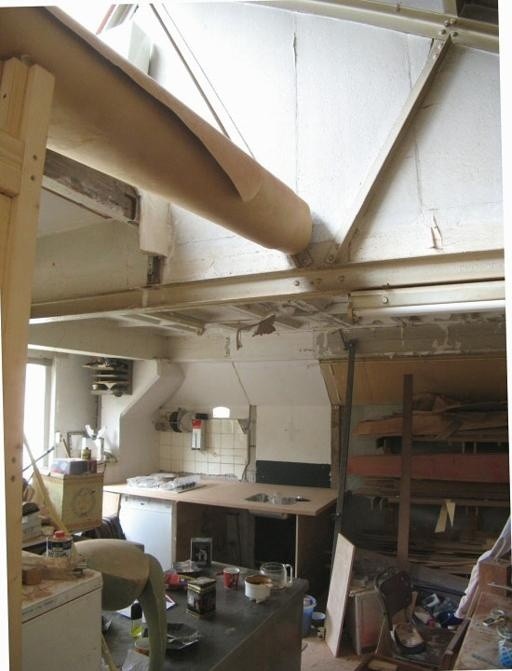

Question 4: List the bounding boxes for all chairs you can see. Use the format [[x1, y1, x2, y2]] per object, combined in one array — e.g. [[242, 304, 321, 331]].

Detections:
[[373, 564, 460, 671]]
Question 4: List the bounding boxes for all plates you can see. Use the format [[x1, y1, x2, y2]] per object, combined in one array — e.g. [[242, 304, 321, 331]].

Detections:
[[170, 411, 194, 433]]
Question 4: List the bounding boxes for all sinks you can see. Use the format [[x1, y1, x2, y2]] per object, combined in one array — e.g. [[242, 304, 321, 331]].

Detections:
[[245, 493, 310, 521]]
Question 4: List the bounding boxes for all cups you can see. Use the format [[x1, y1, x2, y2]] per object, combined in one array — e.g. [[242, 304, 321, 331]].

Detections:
[[135, 638, 149, 658], [260, 562, 293, 589], [245, 575, 271, 603], [223, 567, 241, 590], [89, 461, 97, 474]]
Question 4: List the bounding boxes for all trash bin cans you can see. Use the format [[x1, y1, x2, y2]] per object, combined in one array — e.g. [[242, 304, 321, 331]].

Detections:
[[298, 595, 318, 640]]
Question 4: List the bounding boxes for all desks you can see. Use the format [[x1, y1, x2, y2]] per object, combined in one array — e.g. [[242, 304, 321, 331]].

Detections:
[[94, 550, 309, 671]]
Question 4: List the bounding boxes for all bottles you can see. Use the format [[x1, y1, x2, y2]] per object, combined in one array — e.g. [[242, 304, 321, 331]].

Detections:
[[130, 604, 142, 637], [46, 529, 74, 560], [192, 419, 202, 451], [81, 447, 92, 463]]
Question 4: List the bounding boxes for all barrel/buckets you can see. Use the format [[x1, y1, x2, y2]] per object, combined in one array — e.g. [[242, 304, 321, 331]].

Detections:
[[300, 594, 316, 638]]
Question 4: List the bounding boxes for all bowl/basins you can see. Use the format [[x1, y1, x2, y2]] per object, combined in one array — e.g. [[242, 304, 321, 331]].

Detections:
[[151, 472, 179, 482]]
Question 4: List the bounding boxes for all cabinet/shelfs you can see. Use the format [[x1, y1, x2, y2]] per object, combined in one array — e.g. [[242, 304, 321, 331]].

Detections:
[[122, 496, 224, 573]]
